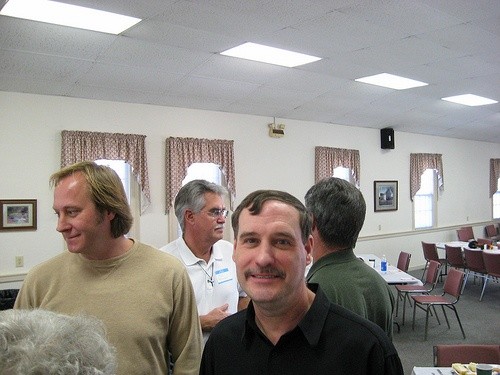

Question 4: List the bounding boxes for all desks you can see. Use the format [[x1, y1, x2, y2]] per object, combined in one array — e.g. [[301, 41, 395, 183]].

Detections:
[[366, 260, 423, 334], [436, 241, 500, 254]]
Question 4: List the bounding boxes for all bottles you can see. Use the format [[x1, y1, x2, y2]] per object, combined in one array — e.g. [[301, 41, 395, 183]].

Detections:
[[369, 256, 375, 269], [380, 254, 388, 275]]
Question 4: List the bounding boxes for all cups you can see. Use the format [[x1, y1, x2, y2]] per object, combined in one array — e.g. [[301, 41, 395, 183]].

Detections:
[[476, 364, 493, 375]]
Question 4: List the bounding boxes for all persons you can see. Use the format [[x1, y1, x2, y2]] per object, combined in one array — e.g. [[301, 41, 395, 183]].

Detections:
[[0, 309, 116, 375], [199, 189, 404, 375], [160, 180, 251, 375], [304, 176, 395, 340], [13, 160, 203, 375]]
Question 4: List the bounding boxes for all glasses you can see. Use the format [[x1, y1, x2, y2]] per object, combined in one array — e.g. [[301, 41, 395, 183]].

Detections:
[[192, 209, 229, 219]]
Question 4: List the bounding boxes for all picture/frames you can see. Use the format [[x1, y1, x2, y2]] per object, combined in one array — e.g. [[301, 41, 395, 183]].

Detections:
[[0, 199, 37, 230], [374, 181, 398, 212]]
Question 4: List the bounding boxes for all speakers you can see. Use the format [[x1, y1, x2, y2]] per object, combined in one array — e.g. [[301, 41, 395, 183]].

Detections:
[[381, 128, 394, 149]]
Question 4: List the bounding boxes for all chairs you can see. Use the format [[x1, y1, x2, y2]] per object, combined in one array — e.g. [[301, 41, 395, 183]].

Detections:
[[396, 225, 500, 367]]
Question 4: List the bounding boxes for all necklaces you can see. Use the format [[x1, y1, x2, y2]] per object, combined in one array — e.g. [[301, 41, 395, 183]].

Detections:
[[197, 262, 214, 287]]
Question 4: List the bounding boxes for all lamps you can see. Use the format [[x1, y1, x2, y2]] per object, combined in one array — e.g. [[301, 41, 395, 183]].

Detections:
[[268, 118, 286, 138]]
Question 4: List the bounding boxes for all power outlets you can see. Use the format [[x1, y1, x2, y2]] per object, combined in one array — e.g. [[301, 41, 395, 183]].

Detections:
[[16, 256, 24, 267]]
